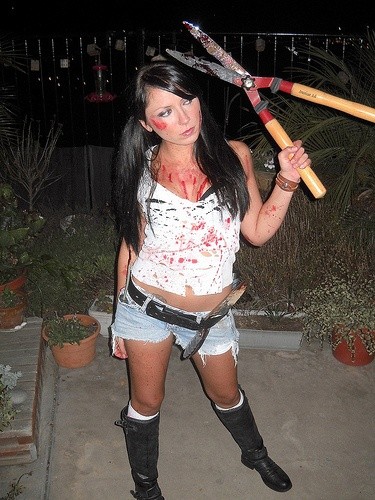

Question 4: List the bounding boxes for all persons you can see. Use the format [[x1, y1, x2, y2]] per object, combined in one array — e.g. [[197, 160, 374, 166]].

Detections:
[[111, 61, 312, 499]]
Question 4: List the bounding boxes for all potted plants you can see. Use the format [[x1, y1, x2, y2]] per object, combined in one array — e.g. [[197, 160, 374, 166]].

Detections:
[[231, 189, 319, 351], [0, 183, 116, 368]]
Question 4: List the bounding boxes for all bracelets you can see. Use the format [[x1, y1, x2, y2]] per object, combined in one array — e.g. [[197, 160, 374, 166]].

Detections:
[[275, 171, 301, 192]]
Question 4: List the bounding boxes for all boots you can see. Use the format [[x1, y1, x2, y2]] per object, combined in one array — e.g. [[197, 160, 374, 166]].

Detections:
[[210, 387, 294, 492], [114, 404, 167, 500]]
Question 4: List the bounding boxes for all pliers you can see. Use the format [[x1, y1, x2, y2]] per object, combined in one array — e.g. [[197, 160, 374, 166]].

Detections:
[[166, 20, 375, 200]]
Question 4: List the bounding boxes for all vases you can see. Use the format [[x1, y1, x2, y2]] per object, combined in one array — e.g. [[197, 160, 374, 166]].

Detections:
[[332, 323, 375, 366]]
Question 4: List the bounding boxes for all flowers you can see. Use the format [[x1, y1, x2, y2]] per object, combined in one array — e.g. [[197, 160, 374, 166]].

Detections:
[[294, 269, 375, 361]]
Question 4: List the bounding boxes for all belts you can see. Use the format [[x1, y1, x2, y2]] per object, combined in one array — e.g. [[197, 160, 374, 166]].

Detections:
[[128, 270, 229, 331]]
